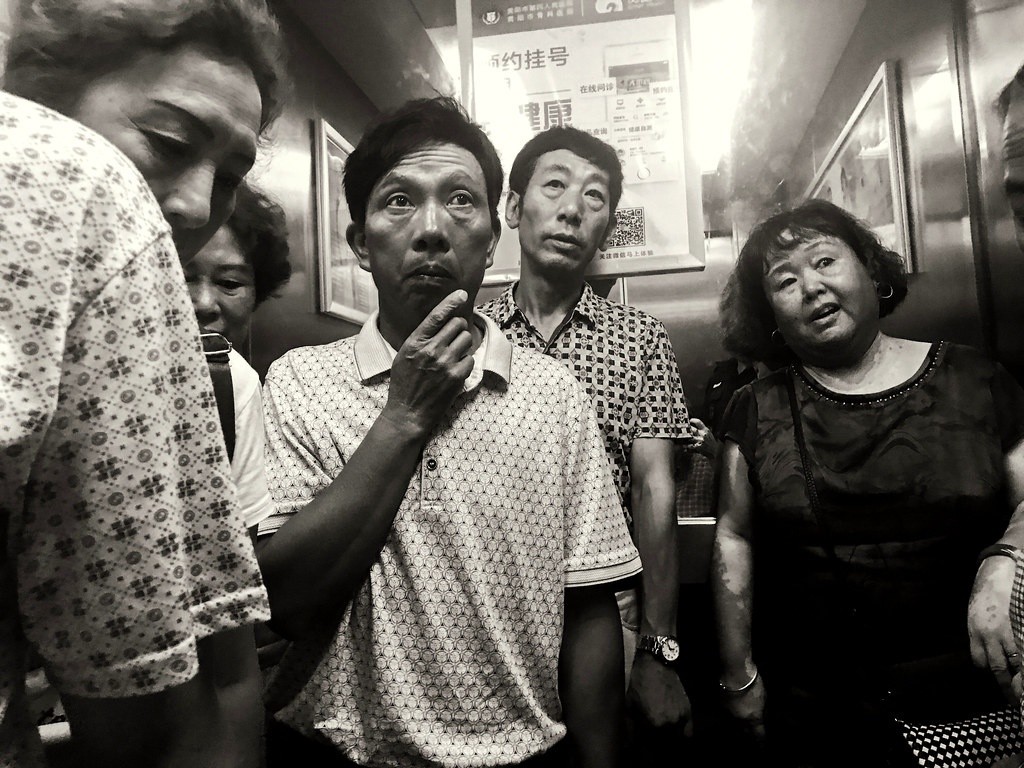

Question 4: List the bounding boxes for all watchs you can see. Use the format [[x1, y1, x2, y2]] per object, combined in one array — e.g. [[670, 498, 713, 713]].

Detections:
[[635, 633, 682, 666]]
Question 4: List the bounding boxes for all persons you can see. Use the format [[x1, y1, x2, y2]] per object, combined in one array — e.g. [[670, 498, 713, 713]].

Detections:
[[182, 179, 292, 349], [676, 356, 758, 518], [475, 125, 694, 768], [256, 97, 644, 768], [997, 67, 1024, 759], [0, 0, 279, 768], [707, 200, 1024, 768]]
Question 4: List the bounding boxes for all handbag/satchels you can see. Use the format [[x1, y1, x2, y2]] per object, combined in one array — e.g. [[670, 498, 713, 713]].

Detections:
[[890, 707, 1024, 768]]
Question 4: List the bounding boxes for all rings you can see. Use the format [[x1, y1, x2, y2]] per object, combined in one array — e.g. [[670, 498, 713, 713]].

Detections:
[[1008, 653, 1019, 658], [694, 428, 698, 434]]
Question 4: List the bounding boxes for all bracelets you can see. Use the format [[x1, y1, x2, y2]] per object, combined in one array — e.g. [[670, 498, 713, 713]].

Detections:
[[719, 664, 759, 694], [976, 543, 1017, 569]]
